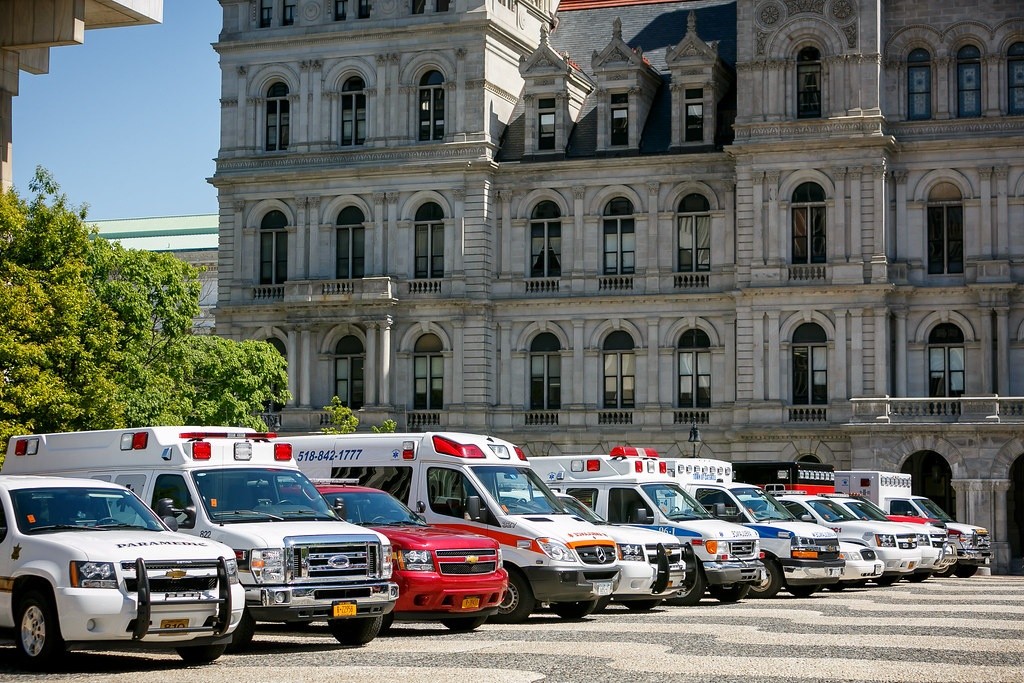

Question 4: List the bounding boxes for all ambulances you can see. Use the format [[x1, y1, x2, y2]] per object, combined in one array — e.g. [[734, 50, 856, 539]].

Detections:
[[529, 448, 760, 607], [0, 426, 399, 654], [272, 431, 626, 624]]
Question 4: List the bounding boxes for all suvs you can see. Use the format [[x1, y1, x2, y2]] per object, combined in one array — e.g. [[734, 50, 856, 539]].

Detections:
[[890, 514, 955, 583], [1, 475, 245, 667], [306, 478, 507, 637], [498, 489, 685, 610]]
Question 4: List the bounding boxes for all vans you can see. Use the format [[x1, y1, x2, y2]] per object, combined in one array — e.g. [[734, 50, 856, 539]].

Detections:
[[656, 457, 846, 601], [834, 469, 994, 578], [730, 461, 921, 583]]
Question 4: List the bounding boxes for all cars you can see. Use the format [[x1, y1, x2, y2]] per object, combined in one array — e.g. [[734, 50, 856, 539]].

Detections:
[[842, 542, 883, 583]]
